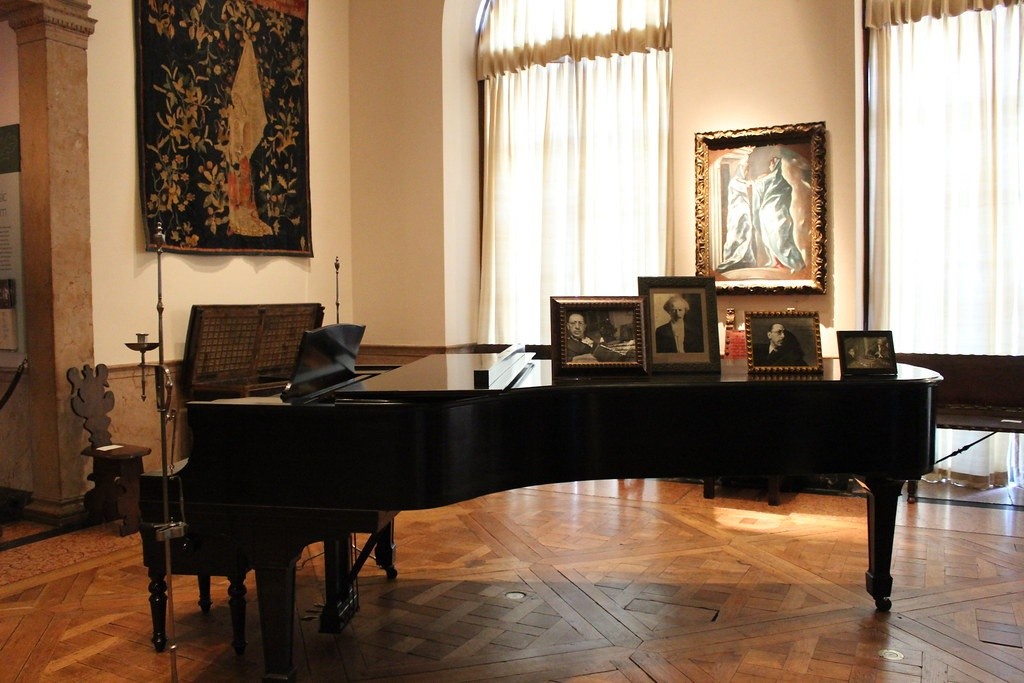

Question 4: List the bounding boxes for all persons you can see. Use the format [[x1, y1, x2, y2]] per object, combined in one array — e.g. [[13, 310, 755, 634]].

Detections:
[[871, 338, 890, 360], [753, 323, 809, 366], [655, 296, 704, 353], [845, 347, 855, 363], [566, 312, 597, 361]]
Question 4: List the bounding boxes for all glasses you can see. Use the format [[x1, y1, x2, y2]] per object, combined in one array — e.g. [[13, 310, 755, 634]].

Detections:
[[771, 330, 787, 335]]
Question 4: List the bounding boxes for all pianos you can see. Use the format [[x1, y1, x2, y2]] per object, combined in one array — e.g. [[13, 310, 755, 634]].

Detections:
[[141, 343, 943, 671]]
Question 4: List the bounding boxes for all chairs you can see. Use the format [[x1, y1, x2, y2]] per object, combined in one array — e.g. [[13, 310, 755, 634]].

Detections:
[[66, 364, 153, 538]]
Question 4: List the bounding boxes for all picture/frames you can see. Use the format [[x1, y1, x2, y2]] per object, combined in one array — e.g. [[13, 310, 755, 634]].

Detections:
[[638, 276, 723, 374], [743, 310, 825, 376], [549, 295, 653, 380], [837, 330, 899, 376], [693, 120, 830, 297]]
